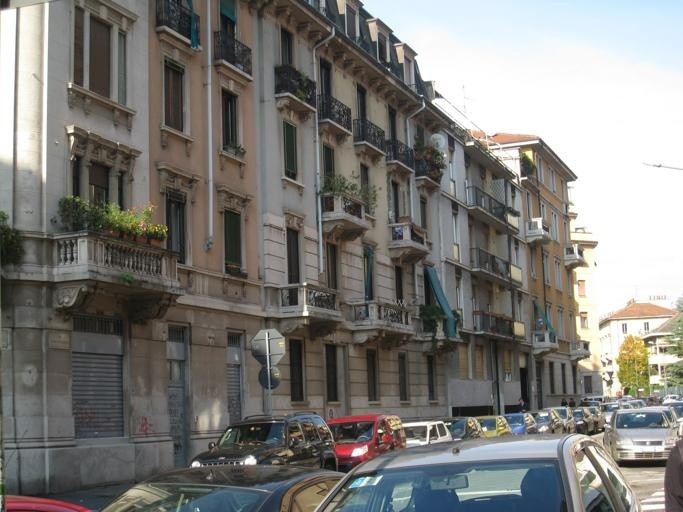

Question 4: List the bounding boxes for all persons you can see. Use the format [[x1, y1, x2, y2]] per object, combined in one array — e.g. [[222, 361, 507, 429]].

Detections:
[[664, 437, 683, 512], [569, 398, 575, 407], [519, 398, 529, 413], [583, 398, 588, 406], [561, 398, 568, 407]]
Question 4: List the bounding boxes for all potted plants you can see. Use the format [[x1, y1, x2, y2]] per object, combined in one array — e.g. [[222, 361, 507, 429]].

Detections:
[[317, 171, 382, 219], [83, 199, 121, 237]]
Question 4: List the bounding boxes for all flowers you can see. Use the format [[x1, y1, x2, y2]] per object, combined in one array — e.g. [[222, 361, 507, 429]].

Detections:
[[122, 196, 169, 240]]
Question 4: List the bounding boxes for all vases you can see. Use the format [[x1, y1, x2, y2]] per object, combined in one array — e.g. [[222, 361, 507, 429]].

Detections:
[[136, 235, 161, 247]]
